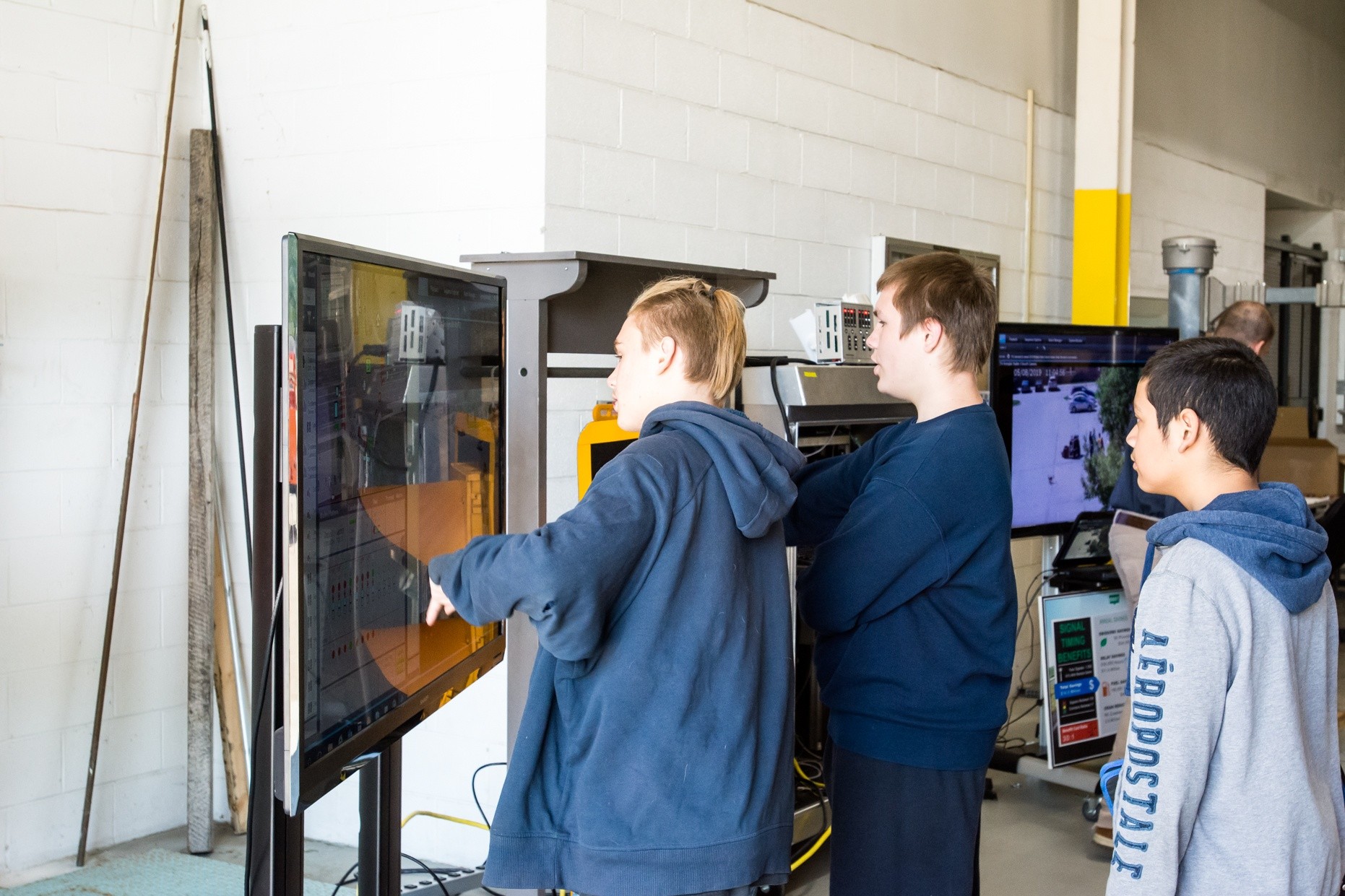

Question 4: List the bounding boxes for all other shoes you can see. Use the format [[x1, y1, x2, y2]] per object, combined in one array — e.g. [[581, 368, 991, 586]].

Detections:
[[1092, 823, 1117, 847]]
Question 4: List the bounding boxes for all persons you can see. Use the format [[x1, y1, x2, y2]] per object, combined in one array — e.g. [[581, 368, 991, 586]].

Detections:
[[780, 250, 1016, 896], [1093, 301, 1277, 846], [423, 278, 808, 896], [1105, 336, 1345, 896]]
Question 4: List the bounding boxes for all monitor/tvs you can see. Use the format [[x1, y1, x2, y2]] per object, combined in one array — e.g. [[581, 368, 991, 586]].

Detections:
[[1036, 587, 1136, 767], [275, 231, 507, 819], [985, 323, 1181, 539]]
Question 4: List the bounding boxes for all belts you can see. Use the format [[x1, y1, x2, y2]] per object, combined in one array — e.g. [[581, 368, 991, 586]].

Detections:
[[1114, 511, 1159, 531]]
